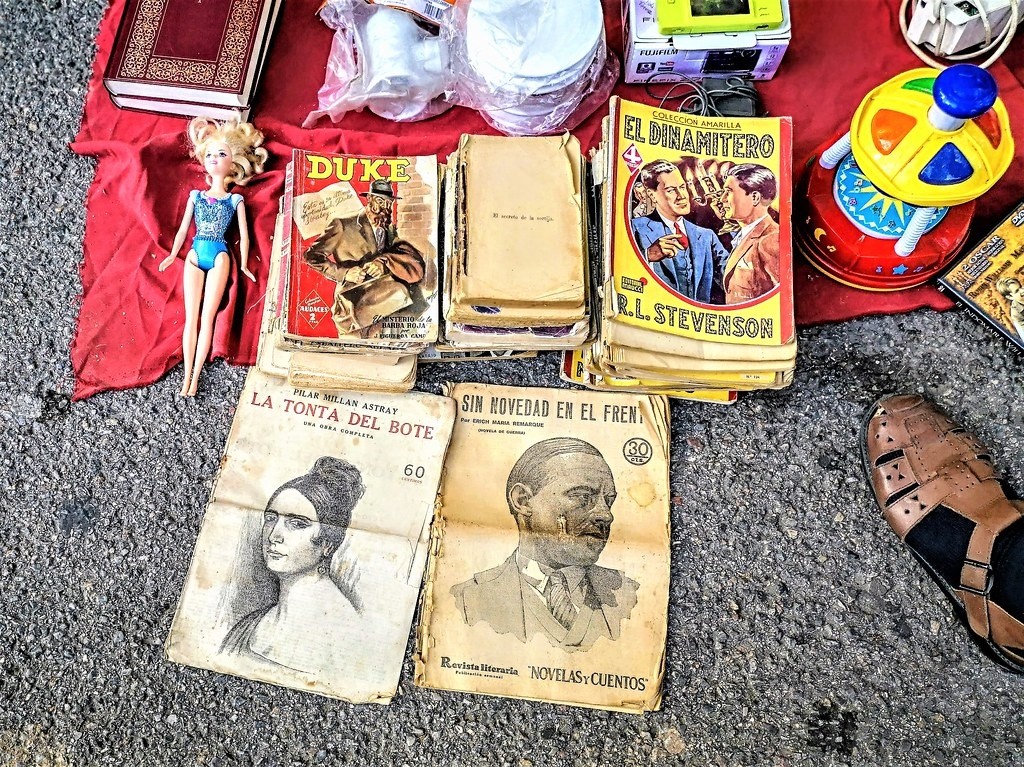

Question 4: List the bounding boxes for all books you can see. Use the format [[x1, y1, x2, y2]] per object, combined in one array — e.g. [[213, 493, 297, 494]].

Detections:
[[556, 91, 795, 400], [934, 200, 1024, 359], [421, 133, 593, 362], [413, 380, 671, 712], [165, 363, 455, 707], [101, 0, 283, 122], [256, 150, 443, 391]]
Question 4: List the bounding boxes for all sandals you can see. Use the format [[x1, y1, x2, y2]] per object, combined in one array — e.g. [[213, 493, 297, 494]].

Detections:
[[857, 391, 1024, 678]]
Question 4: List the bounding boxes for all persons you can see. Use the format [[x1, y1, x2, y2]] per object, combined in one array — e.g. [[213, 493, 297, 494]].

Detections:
[[158, 105, 267, 397], [858, 392, 1024, 674]]
